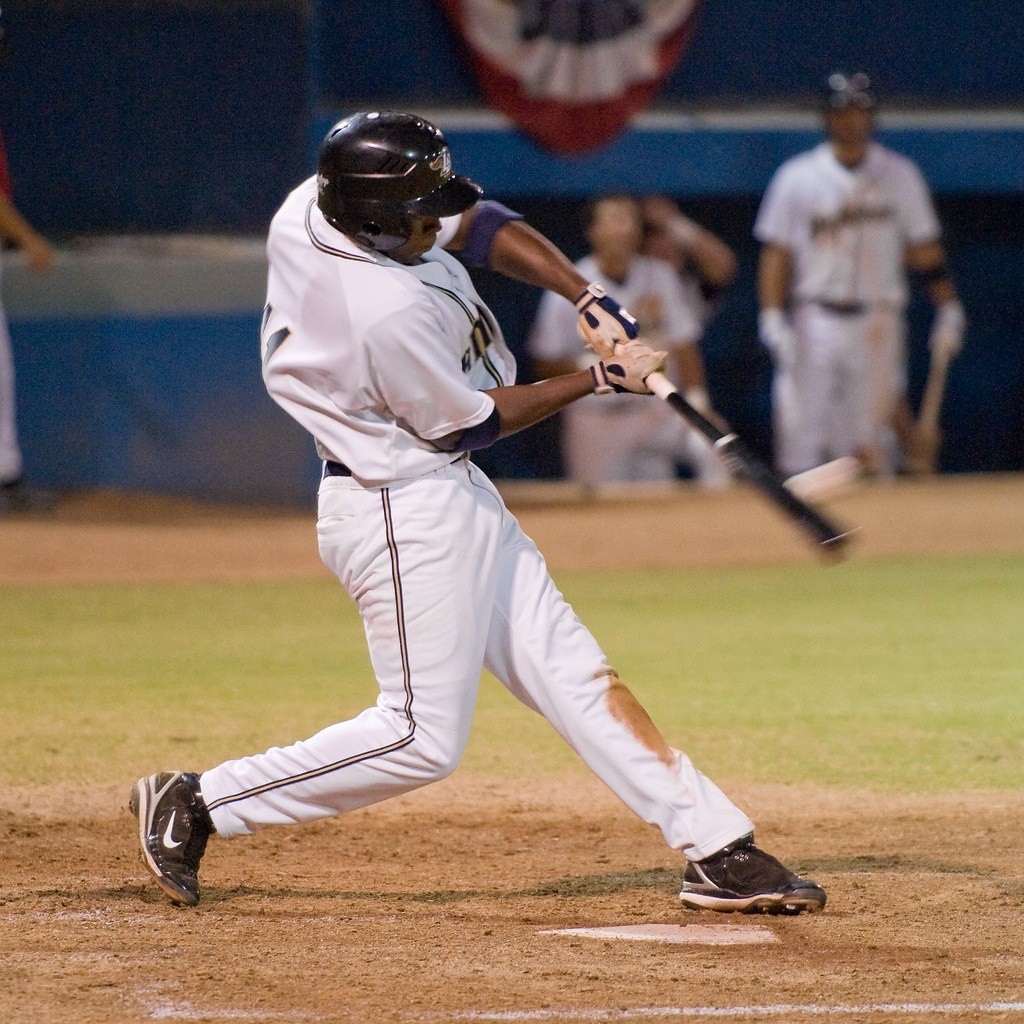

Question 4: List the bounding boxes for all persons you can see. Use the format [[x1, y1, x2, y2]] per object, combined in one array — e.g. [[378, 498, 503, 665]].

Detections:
[[528, 195, 733, 481], [127, 111, 826, 914], [1, 132, 56, 506], [753, 71, 964, 477]]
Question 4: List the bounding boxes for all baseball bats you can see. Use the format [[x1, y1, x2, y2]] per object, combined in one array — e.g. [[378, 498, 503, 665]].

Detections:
[[907, 348, 950, 476], [572, 320, 852, 560]]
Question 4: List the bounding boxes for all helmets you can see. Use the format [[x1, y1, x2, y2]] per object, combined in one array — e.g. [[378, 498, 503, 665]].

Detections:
[[317, 112, 483, 251]]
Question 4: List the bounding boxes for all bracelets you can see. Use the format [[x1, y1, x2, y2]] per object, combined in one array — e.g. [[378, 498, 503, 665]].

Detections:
[[670, 216, 696, 242]]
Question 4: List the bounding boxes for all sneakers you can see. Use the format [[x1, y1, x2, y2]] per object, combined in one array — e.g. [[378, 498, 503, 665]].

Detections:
[[129, 771, 217, 907], [679, 835, 827, 912]]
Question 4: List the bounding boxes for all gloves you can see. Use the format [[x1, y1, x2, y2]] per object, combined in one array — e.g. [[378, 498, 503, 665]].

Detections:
[[590, 340, 668, 397], [574, 280, 641, 360]]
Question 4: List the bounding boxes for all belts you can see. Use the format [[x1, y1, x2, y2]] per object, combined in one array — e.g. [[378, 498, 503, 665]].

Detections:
[[323, 460, 352, 479]]
